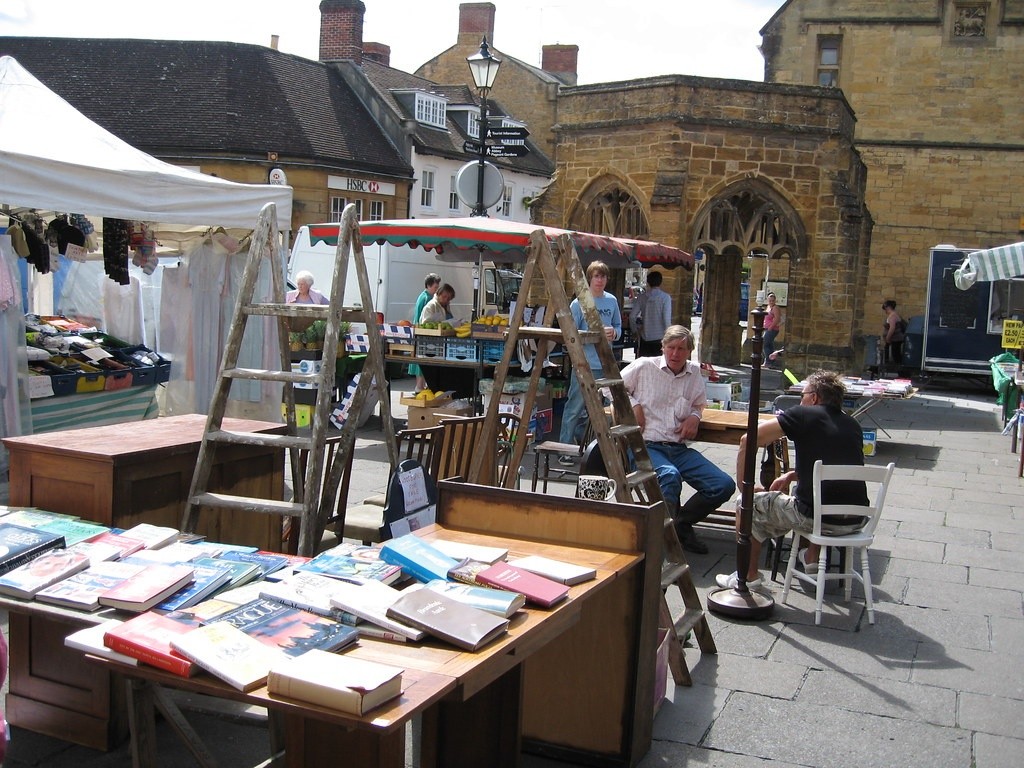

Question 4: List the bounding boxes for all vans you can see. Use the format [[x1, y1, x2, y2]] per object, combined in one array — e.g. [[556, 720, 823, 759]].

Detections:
[[284, 224, 534, 378], [692, 291, 699, 315], [696, 282, 750, 322]]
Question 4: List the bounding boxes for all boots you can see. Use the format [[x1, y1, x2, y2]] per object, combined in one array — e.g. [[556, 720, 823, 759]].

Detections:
[[674, 493, 709, 554], [665, 503, 680, 522]]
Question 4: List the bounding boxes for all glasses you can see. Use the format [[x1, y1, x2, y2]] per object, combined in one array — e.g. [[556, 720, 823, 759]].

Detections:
[[591, 274, 607, 279], [801, 391, 815, 399]]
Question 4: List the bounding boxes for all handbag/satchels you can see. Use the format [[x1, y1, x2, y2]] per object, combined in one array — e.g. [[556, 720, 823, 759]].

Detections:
[[899, 318, 907, 335], [700, 361, 718, 382]]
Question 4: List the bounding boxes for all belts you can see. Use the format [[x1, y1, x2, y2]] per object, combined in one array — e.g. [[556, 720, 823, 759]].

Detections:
[[645, 441, 685, 446]]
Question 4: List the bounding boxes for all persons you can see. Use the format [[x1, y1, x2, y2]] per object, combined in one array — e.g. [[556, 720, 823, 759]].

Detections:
[[713, 368, 871, 591], [629, 271, 672, 358], [617, 322, 735, 556], [553, 260, 622, 465], [761, 292, 781, 368], [285, 271, 329, 304], [404, 273, 457, 391], [882, 301, 907, 365]]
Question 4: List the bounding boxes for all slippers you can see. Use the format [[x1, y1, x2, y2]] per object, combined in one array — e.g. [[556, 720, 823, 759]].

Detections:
[[411, 391, 419, 396]]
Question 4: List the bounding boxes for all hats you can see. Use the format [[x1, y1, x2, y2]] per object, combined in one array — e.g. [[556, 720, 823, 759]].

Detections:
[[882, 301, 896, 310]]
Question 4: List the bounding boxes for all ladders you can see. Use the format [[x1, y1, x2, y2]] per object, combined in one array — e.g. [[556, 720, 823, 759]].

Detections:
[[177, 203, 408, 559], [467, 227, 724, 684]]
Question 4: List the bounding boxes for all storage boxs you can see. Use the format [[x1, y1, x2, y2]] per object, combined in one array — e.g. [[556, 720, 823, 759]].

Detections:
[[862, 427, 877, 457], [280, 312, 562, 454], [17, 315, 171, 402]]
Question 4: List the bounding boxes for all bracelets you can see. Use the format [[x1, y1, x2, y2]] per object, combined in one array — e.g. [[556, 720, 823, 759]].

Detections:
[[690, 411, 703, 420]]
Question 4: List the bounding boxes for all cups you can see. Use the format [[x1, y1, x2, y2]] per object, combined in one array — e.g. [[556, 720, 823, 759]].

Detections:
[[604, 327, 613, 340], [579, 475, 617, 501]]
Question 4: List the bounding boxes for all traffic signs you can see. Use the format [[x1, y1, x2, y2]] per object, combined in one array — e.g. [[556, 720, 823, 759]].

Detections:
[[463, 127, 531, 158]]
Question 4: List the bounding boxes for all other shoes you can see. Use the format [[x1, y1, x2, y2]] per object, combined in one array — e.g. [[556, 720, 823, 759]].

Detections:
[[761, 363, 775, 369]]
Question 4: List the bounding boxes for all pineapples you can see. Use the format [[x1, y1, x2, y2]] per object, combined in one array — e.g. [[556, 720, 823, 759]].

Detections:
[[289, 319, 350, 358]]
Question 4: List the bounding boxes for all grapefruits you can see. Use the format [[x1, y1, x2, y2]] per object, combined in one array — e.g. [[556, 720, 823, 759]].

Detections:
[[415, 390, 444, 400]]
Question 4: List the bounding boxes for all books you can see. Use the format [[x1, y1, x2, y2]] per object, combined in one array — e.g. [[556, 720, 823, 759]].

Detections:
[[0, 507, 596, 716], [784, 374, 914, 399]]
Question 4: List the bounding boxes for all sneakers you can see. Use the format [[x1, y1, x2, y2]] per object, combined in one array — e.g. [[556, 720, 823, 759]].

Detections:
[[797, 548, 818, 574], [557, 455, 574, 466], [715, 571, 762, 593]]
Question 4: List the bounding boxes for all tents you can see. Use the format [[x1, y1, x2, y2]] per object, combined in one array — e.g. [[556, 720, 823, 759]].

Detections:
[[0, 56, 298, 467]]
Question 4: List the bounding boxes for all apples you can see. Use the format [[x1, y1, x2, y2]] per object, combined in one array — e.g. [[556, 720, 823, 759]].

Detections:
[[420, 320, 453, 329], [398, 320, 411, 327]]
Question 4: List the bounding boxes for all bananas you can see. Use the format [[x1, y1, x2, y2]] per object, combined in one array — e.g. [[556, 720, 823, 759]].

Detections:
[[453, 322, 471, 338]]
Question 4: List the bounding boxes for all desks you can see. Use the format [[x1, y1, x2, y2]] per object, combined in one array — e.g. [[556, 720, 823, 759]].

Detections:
[[785, 386, 919, 438], [1, 412, 293, 550], [600, 405, 786, 580], [0, 506, 304, 751], [343, 352, 558, 416], [65, 528, 635, 768]]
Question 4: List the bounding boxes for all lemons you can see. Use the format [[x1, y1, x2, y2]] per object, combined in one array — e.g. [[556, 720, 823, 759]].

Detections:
[[476, 315, 511, 325]]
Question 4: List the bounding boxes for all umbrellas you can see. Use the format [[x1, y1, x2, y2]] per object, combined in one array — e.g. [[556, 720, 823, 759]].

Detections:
[[306, 213, 697, 325]]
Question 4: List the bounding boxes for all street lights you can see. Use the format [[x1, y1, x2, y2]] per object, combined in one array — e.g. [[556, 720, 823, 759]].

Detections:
[[464, 35, 503, 217]]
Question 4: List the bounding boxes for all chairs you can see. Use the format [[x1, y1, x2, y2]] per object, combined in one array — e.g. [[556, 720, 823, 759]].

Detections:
[[317, 390, 650, 554], [767, 435, 898, 625]]
